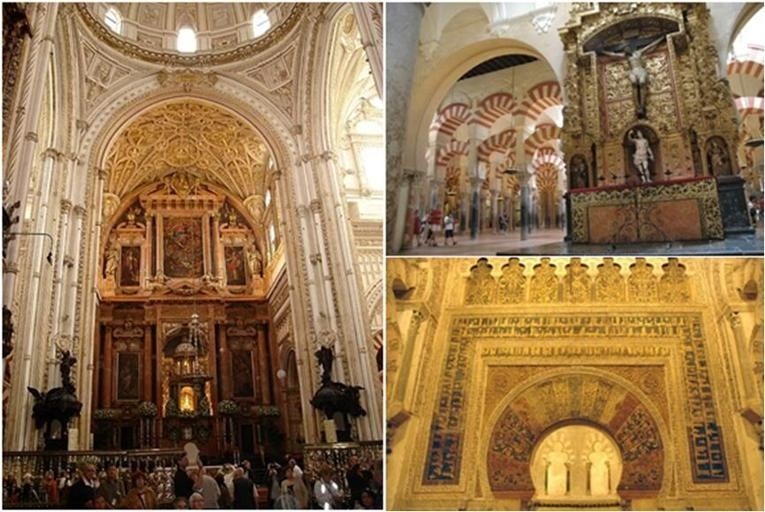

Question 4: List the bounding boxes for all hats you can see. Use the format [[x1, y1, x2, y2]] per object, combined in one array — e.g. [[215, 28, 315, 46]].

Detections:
[[173, 452, 187, 463]]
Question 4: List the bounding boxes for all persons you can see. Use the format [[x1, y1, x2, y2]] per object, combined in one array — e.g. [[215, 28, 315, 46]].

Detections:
[[225, 248, 241, 282], [594, 34, 670, 117], [246, 242, 264, 278], [746, 193, 764, 228], [405, 200, 459, 248], [627, 128, 657, 183], [499, 208, 510, 235], [104, 243, 120, 279], [124, 249, 139, 284]]
